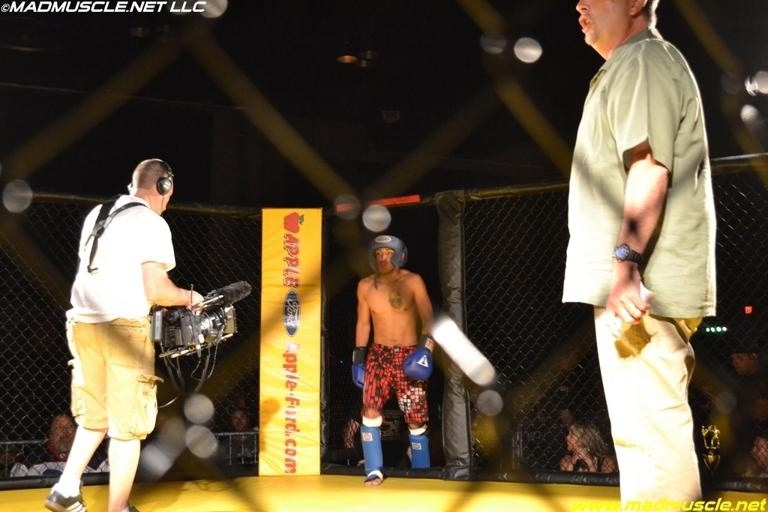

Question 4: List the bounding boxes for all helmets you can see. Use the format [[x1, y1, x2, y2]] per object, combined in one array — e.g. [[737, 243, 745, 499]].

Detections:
[[366, 235, 409, 271]]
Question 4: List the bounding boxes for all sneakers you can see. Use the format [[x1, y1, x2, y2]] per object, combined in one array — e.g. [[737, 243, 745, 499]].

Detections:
[[43, 479, 88, 512]]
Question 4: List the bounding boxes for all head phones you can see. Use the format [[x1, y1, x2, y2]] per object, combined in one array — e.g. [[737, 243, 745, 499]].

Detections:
[[126, 158, 174, 195]]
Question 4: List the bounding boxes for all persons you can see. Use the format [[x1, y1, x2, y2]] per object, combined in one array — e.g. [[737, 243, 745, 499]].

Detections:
[[43, 158, 206, 511], [348, 231, 436, 488], [555, 1, 722, 512], [0, 339, 767, 486]]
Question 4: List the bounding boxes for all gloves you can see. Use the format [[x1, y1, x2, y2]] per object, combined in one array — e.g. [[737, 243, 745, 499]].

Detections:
[[402, 336, 435, 380], [353, 348, 368, 388]]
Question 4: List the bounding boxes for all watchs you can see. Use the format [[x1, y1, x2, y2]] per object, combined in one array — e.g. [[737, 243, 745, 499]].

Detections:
[[609, 243, 644, 266]]
